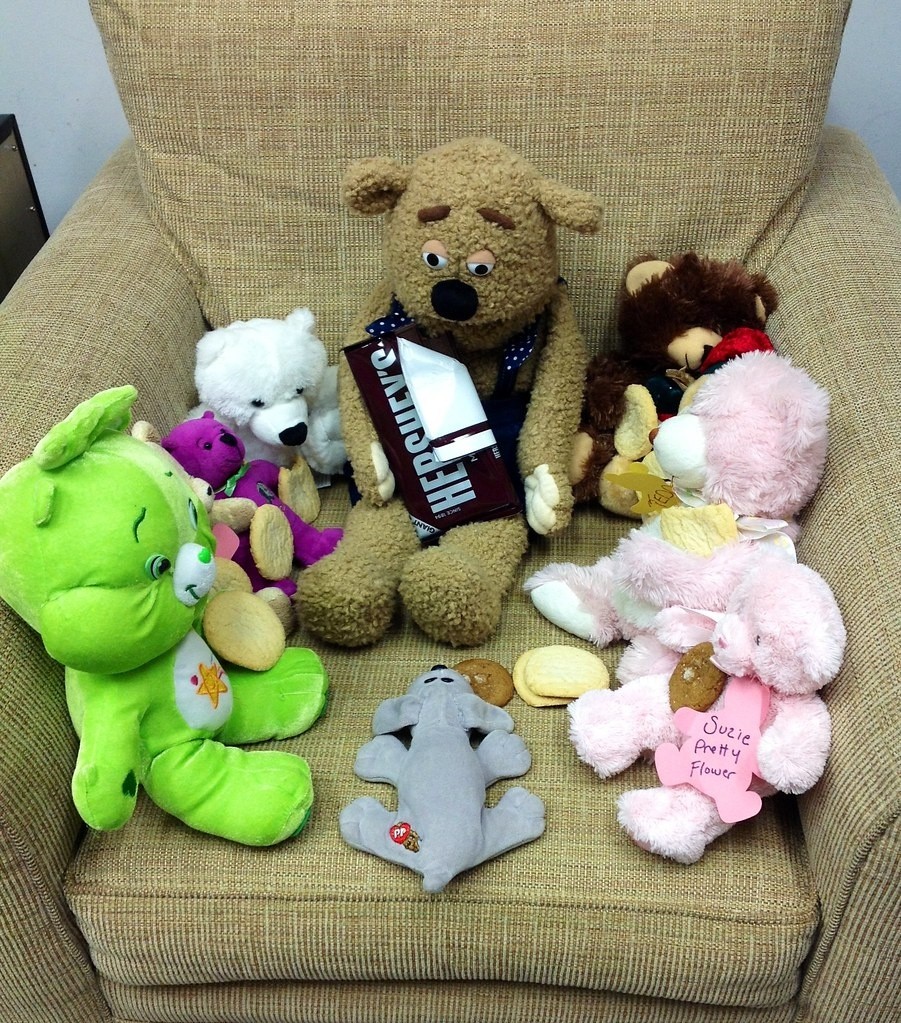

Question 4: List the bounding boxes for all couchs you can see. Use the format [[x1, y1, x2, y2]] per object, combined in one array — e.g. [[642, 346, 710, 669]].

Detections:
[[0, 0, 901, 1023]]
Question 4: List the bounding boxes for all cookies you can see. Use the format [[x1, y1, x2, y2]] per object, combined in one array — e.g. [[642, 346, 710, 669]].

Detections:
[[512, 644, 610, 707], [203, 591, 286, 671], [448, 659, 514, 707], [277, 458, 322, 524], [660, 503, 737, 553], [207, 557, 254, 596], [614, 384, 659, 460], [249, 503, 295, 580], [669, 642, 725, 712]]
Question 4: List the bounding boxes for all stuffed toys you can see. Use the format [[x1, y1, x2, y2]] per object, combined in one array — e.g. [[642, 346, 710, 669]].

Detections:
[[297, 137, 604, 646], [0, 252, 847, 889]]
